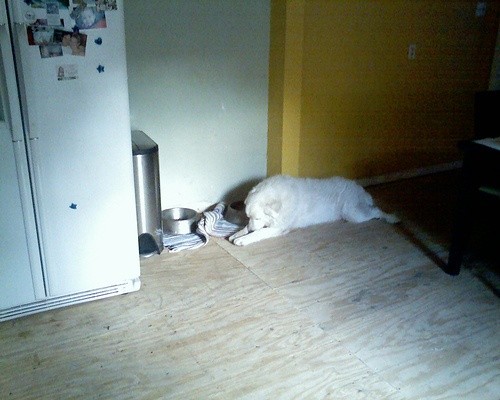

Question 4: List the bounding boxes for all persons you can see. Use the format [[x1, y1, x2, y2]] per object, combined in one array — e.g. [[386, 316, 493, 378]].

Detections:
[[55, 32, 73, 56], [70, 34, 85, 55], [78, 8, 106, 30]]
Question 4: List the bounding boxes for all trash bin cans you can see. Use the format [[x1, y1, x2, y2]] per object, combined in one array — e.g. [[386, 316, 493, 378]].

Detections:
[[131, 129, 164, 258]]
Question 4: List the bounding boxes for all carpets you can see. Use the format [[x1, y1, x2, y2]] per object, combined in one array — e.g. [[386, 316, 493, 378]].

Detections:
[[161, 201, 245, 254]]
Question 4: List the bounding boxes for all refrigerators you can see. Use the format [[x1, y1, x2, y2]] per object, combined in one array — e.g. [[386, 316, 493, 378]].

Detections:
[[0, 0, 141, 323]]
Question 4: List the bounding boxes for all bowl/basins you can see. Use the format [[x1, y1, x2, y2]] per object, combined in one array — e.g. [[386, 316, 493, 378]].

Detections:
[[159, 209, 201, 235]]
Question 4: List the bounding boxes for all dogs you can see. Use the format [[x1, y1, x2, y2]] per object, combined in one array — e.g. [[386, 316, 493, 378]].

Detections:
[[227, 174, 402, 246]]
